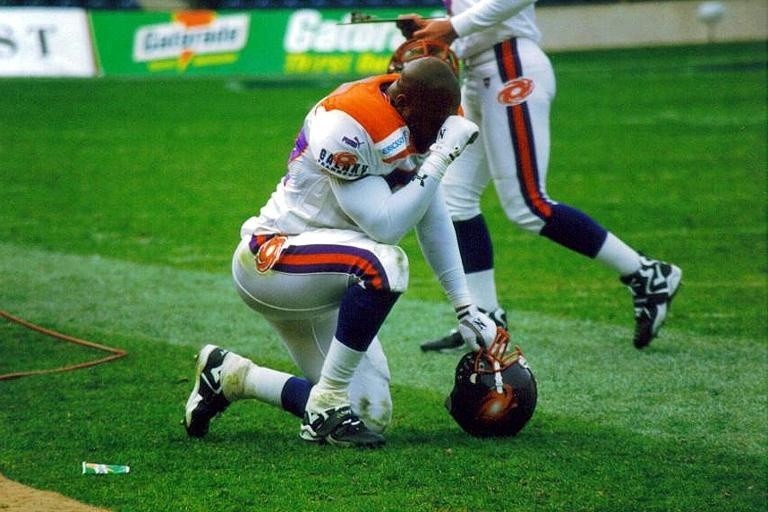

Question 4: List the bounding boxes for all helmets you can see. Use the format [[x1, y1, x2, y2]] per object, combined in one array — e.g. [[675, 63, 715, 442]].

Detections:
[[444, 348, 538, 440]]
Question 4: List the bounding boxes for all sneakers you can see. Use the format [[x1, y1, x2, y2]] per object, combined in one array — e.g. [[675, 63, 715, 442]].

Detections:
[[621, 248, 682, 350], [420, 306, 510, 354], [179, 342, 251, 437], [299, 386, 387, 450]]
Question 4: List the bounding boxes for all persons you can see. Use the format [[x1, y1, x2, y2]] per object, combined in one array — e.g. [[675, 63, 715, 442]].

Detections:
[[395, 1, 686, 359], [182, 58, 499, 445]]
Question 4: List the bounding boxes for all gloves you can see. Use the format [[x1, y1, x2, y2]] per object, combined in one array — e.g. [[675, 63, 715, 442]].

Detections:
[[430, 114, 481, 165], [455, 304, 498, 354]]
